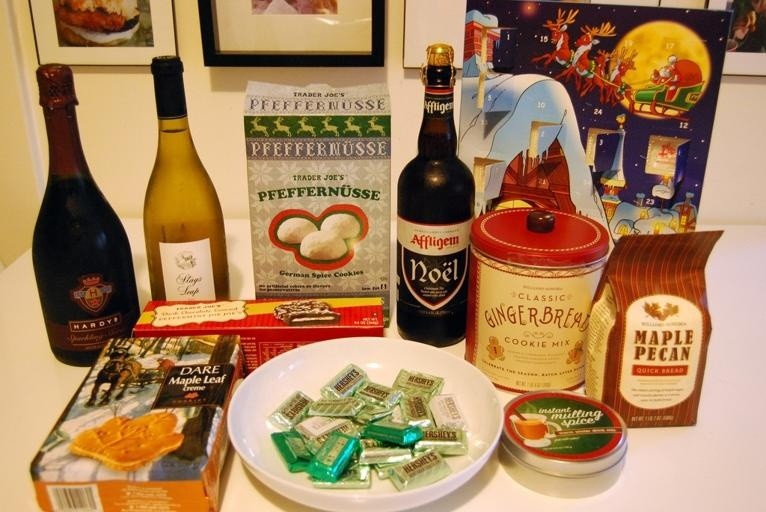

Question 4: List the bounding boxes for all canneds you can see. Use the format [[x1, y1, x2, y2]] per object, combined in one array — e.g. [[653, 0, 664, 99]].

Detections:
[[464, 207, 613, 395]]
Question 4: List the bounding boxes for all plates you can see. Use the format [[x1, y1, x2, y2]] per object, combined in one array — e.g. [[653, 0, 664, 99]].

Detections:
[[226, 336, 503, 512]]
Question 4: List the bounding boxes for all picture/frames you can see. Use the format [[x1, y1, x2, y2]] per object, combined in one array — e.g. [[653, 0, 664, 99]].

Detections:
[[198, 0, 390, 71], [25, 1, 182, 74]]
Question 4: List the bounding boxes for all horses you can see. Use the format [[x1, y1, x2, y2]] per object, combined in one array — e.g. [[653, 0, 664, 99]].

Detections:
[[87, 346, 142, 408]]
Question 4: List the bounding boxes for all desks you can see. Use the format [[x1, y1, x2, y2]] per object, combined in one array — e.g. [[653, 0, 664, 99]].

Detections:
[[1, 214, 766, 512]]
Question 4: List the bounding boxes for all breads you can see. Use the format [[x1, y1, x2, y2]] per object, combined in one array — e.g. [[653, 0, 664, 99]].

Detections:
[[276, 213, 361, 261]]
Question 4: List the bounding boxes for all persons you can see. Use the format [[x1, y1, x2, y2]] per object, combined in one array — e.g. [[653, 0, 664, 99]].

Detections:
[[731, 0, 765, 52]]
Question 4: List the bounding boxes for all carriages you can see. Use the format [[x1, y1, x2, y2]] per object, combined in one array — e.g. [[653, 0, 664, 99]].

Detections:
[[87, 344, 176, 409]]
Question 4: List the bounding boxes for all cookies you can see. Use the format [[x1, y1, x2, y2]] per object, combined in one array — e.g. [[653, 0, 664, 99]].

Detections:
[[68, 412, 184, 472]]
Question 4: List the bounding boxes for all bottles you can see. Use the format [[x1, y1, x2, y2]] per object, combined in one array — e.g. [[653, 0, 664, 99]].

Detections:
[[28, 62, 140, 369], [395, 45, 471, 345], [140, 52, 231, 303]]
